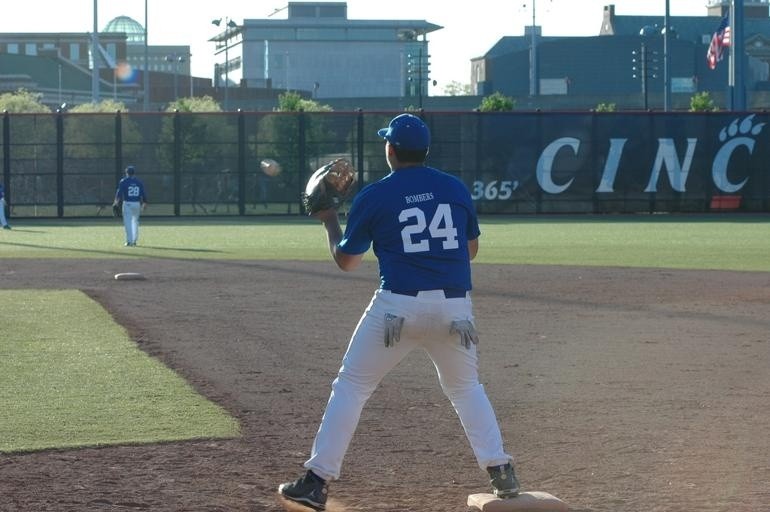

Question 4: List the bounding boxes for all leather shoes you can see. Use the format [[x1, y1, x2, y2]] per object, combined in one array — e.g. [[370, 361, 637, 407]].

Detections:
[[450, 320, 479, 349], [384, 314, 404, 347]]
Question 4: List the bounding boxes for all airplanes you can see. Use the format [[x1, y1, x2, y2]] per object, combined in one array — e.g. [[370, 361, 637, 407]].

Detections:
[[300, 158, 356, 216]]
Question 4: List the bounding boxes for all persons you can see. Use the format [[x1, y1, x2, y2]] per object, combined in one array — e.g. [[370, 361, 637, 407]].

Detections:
[[279, 112, 521, 511], [114, 166, 147, 246], [0, 175, 11, 230]]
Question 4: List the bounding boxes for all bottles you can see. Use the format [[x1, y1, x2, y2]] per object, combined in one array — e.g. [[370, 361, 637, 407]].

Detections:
[[126, 167, 134, 174], [378, 114, 430, 150]]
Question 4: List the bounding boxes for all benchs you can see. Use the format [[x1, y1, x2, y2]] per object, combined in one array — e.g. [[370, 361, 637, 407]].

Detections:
[[707, 7, 730, 71]]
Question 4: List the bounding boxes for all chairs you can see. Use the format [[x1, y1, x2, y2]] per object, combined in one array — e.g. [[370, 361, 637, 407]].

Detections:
[[4, 225, 10, 229], [487, 464, 520, 497], [125, 242, 136, 246], [278, 470, 328, 509]]
